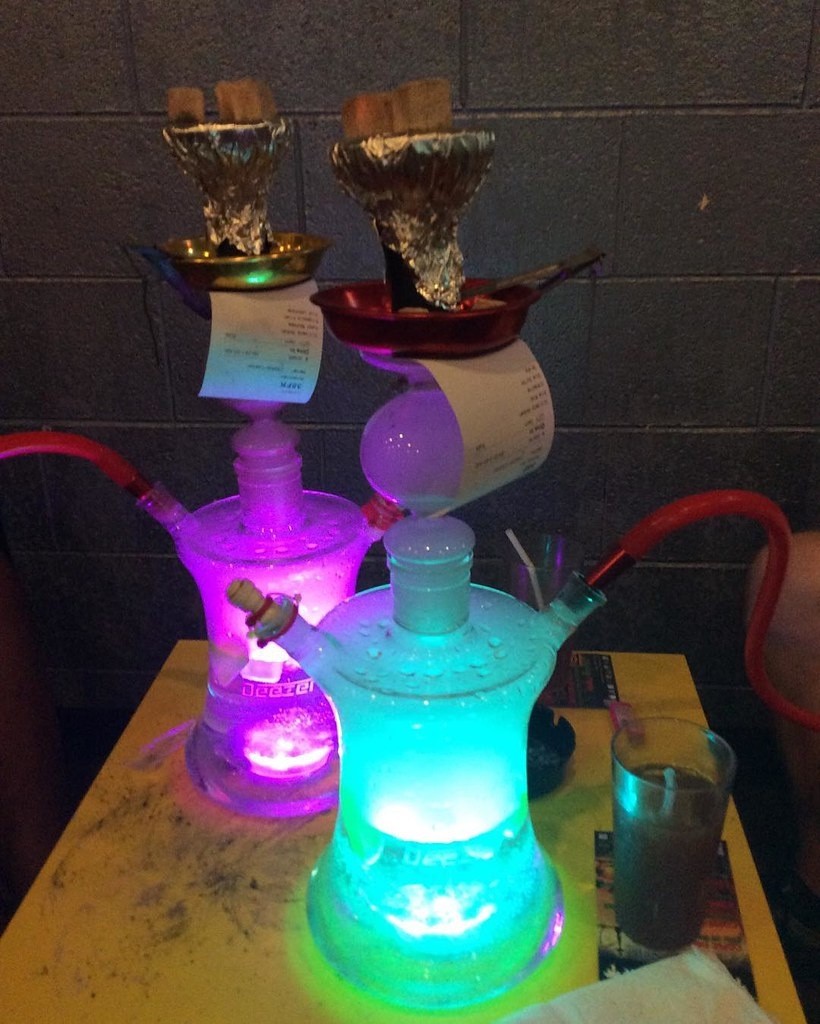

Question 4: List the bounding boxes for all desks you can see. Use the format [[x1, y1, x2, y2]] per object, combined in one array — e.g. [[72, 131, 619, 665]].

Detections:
[[0, 639, 807, 1024]]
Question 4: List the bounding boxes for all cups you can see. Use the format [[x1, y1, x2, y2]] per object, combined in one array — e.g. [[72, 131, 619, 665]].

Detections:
[[514, 563, 579, 612], [612, 717, 733, 952]]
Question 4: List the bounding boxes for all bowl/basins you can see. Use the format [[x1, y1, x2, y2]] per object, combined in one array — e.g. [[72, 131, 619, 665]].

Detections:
[[157, 234, 331, 289], [307, 282, 542, 358]]
